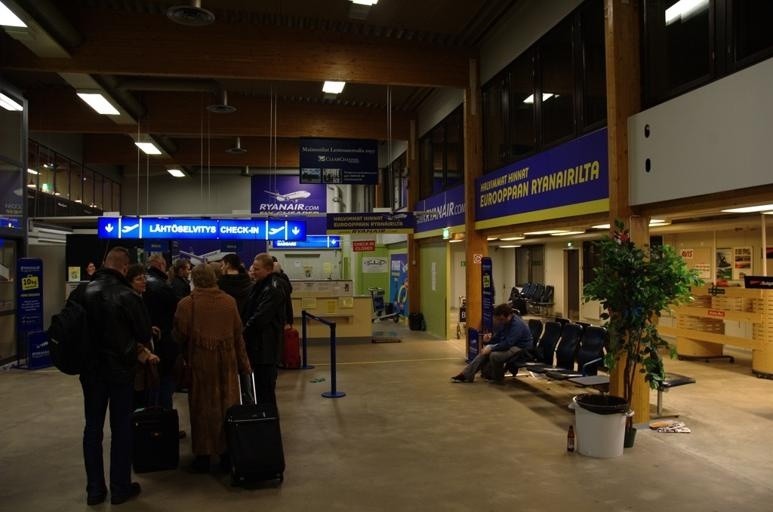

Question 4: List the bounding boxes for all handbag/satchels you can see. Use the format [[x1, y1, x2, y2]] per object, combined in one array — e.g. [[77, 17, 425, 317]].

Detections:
[[160, 340, 193, 376], [48, 282, 88, 374]]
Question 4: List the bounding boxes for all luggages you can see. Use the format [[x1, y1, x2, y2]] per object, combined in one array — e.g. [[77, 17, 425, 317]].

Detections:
[[224, 372, 285, 490], [133, 409, 179, 474], [281, 325, 301, 368]]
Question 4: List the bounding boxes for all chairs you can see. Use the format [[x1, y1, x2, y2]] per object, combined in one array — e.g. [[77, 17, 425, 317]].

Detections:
[[651, 369, 697, 423], [506, 317, 611, 396], [516, 280, 555, 317]]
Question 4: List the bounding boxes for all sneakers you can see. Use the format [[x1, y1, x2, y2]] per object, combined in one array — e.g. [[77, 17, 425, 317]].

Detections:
[[87, 486, 107, 504], [451, 375, 474, 383], [111, 482, 141, 504]]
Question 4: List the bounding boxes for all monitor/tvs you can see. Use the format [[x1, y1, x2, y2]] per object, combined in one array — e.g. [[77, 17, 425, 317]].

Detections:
[[298, 136, 380, 186]]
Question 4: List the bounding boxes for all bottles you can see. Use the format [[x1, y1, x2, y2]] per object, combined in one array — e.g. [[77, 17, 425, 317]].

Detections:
[[568, 425, 575, 452]]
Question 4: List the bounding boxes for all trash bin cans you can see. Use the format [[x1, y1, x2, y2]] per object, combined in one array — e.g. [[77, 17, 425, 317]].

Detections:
[[408, 313, 423, 330], [572, 393, 629, 459]]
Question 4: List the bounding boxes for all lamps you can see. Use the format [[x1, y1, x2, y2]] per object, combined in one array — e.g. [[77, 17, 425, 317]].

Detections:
[[164, 164, 187, 179], [135, 138, 163, 155], [76, 88, 120, 116], [0, 0, 30, 28], [0, 87, 24, 112]]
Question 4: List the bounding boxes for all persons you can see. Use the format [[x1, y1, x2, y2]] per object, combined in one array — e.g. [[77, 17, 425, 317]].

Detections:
[[451, 304, 535, 383], [79, 245, 295, 505], [738, 272, 746, 281], [397, 279, 409, 317]]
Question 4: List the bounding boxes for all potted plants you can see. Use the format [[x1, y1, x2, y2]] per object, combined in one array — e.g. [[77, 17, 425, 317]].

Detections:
[[580, 216, 729, 448]]
